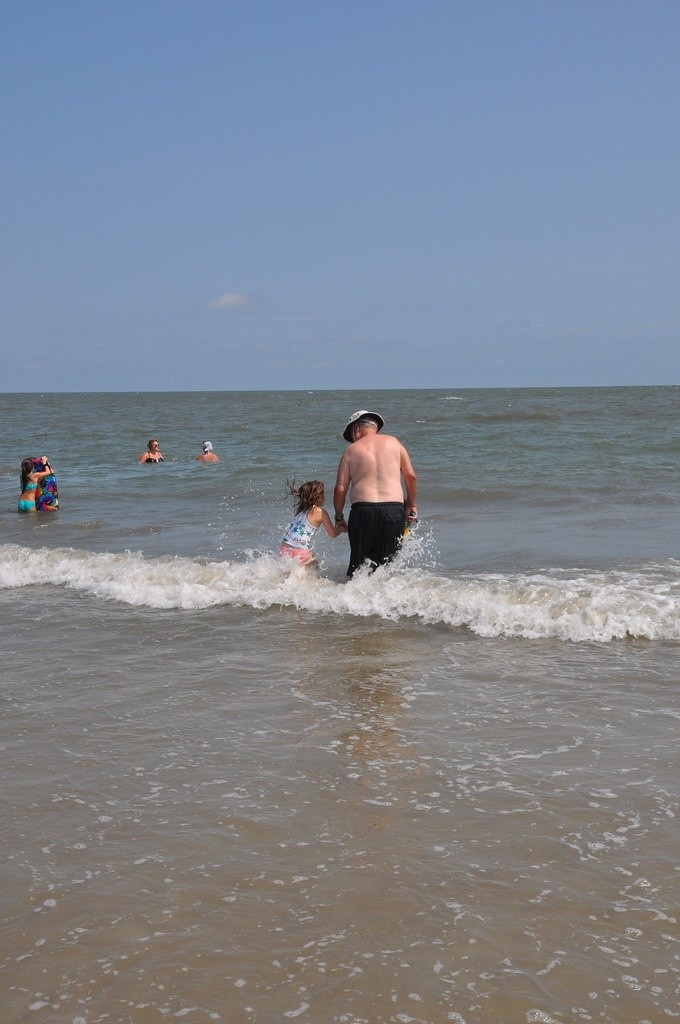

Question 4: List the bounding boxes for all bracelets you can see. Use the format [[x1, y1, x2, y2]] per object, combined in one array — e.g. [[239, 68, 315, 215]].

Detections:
[[335, 515, 344, 520]]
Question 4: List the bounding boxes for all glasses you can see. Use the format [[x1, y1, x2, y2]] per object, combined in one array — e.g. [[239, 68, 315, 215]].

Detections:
[[151, 445, 159, 447]]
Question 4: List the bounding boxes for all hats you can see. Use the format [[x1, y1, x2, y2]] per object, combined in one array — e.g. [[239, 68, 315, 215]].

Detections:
[[343, 410, 385, 442]]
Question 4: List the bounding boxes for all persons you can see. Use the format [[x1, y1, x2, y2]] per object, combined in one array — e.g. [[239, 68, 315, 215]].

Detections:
[[333, 410, 417, 580], [140, 439, 165, 464], [197, 441, 219, 463], [18, 456, 60, 513], [279, 480, 343, 577]]
